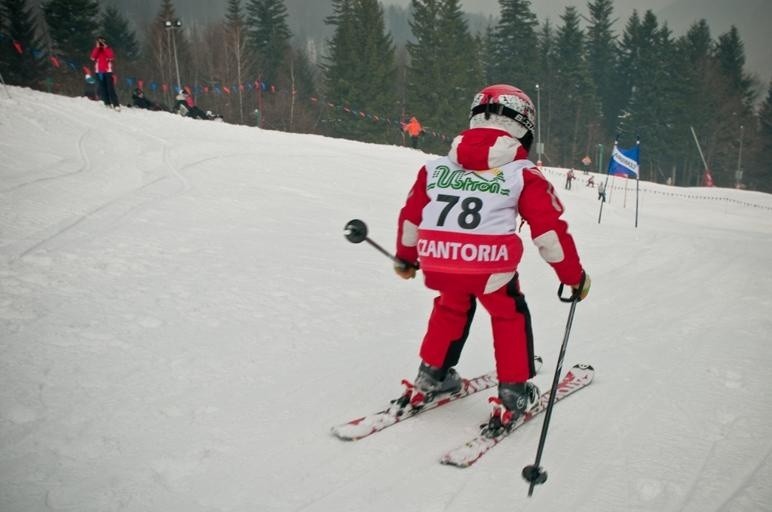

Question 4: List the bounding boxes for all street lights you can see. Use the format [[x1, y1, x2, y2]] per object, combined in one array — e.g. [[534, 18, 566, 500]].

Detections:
[[164, 18, 184, 95], [532, 82, 543, 162]]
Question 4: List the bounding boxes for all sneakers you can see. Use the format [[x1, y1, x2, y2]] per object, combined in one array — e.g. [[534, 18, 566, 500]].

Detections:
[[414, 366, 462, 392], [393, 257, 416, 279], [552, 269, 592, 304], [499, 382, 541, 408]]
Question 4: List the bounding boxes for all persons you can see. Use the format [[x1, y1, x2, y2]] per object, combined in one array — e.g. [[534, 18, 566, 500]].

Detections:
[[403, 116, 422, 149], [173, 89, 223, 120], [582, 154, 592, 175], [565, 168, 576, 190], [89, 37, 121, 112], [598, 182, 606, 202], [395, 85, 589, 430], [585, 176, 594, 187], [132, 88, 172, 113]]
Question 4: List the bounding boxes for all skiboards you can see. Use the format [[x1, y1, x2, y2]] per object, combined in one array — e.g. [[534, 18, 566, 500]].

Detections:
[[329, 354, 595, 467]]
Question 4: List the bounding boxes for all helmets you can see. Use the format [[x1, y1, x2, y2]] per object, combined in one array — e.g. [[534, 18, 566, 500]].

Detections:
[[468, 82, 537, 151]]
